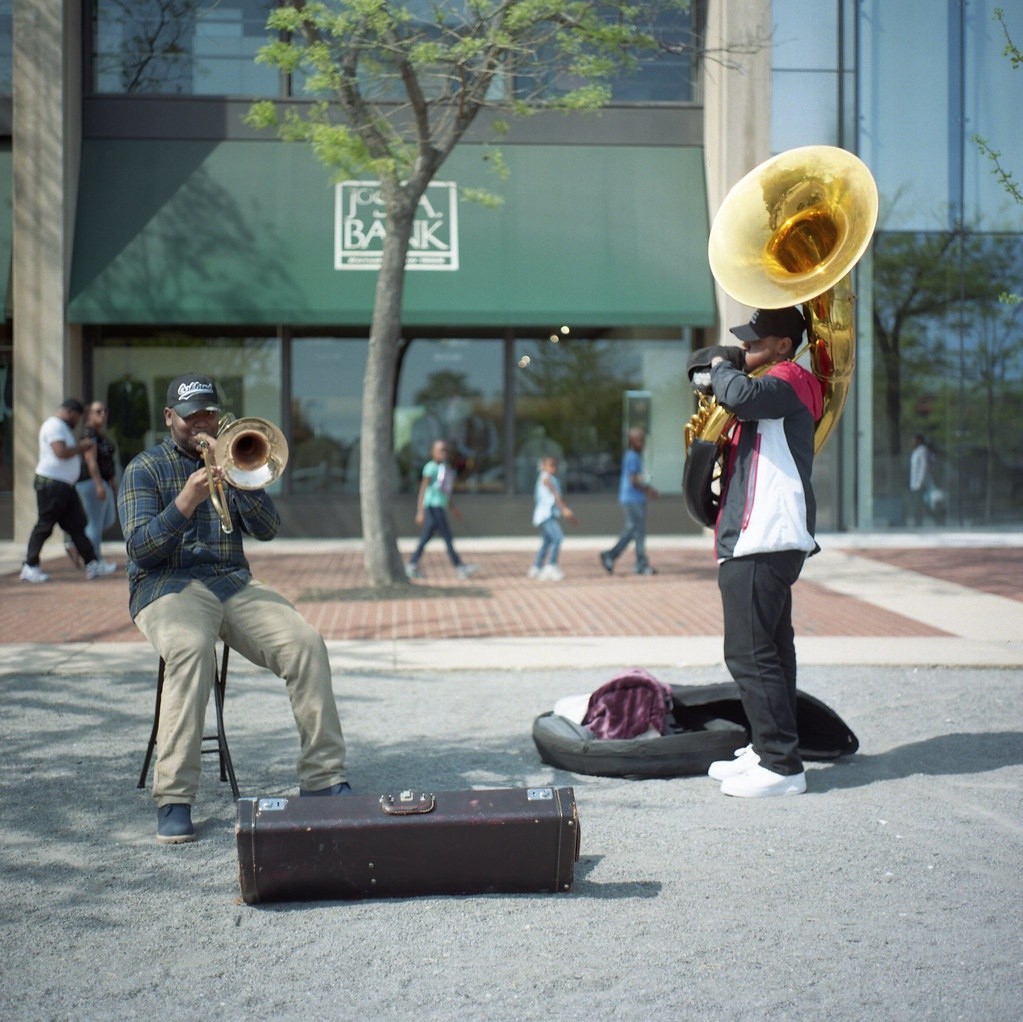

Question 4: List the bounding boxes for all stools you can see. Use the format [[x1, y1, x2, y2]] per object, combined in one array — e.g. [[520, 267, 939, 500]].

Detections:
[[136, 633, 242, 807]]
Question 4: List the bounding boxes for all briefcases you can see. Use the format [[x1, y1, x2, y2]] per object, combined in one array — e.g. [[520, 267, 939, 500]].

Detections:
[[234, 782, 581, 904]]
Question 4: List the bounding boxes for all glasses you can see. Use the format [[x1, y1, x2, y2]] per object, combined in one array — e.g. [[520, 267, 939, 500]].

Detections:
[[93, 409, 108, 415]]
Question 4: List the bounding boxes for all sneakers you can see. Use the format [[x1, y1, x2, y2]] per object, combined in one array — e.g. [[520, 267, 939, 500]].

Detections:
[[722, 760, 807, 798], [708, 742, 764, 781]]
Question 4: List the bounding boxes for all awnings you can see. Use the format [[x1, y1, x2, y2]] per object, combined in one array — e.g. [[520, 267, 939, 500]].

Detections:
[[65, 140, 718, 324]]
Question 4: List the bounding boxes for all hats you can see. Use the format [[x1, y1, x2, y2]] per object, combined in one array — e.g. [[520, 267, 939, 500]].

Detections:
[[729, 305, 806, 343], [166, 373, 223, 419]]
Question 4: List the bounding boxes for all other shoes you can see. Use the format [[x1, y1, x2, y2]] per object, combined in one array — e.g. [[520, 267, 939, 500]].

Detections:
[[84, 561, 116, 579], [298, 782, 351, 799], [20, 563, 48, 583], [156, 796, 197, 844], [529, 564, 565, 583], [407, 563, 423, 579], [637, 566, 657, 575], [458, 566, 476, 579], [601, 553, 613, 575]]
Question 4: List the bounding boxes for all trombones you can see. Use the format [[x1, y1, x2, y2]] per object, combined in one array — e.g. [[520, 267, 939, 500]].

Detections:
[[193, 411, 290, 535]]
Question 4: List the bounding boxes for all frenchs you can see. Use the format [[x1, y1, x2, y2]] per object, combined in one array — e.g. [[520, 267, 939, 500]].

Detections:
[[679, 143, 880, 530]]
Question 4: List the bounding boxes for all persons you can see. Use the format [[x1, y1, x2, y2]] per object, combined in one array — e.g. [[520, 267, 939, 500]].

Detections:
[[527, 454, 575, 581], [686, 306, 855, 799], [909, 435, 930, 528], [64, 398, 115, 571], [18, 397, 117, 585], [405, 438, 479, 579], [117, 371, 353, 845], [600, 427, 661, 577]]
